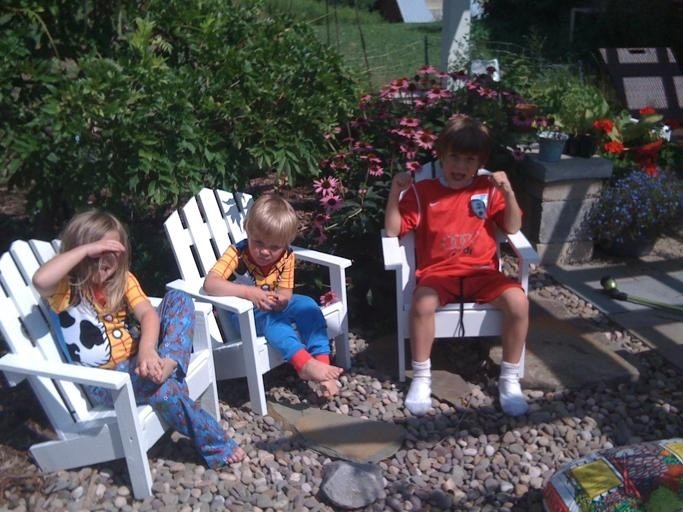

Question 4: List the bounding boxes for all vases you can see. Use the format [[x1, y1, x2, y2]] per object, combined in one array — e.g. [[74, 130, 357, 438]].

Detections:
[[535, 131, 567, 160]]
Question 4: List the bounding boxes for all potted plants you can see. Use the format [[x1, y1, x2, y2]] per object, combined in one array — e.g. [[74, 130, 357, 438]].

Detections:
[[562, 79, 607, 157]]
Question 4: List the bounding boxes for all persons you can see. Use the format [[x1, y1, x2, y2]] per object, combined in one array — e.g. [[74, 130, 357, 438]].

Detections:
[[202, 193, 344, 399], [382, 113, 530, 417], [32, 207, 246, 466]]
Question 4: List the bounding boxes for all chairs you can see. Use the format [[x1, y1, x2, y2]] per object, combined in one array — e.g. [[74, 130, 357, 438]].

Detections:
[[162, 184, 352, 418], [0, 237, 222, 498], [383, 160, 535, 372]]
